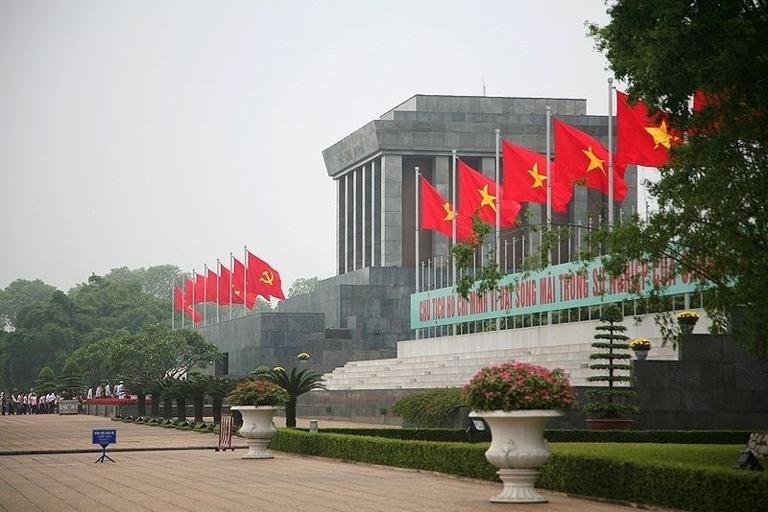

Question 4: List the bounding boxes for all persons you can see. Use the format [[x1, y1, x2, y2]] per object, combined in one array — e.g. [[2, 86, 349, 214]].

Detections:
[[0, 381, 131, 415]]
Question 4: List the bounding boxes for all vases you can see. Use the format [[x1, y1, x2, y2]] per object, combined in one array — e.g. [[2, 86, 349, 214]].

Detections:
[[299, 360, 306, 364], [468, 410, 567, 502], [634, 350, 649, 360], [230, 405, 286, 458], [679, 324, 695, 334]]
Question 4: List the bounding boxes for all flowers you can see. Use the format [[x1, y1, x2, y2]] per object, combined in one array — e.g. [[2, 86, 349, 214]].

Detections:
[[458, 359, 580, 413], [676, 312, 699, 324], [226, 377, 290, 408], [297, 352, 310, 360], [629, 337, 653, 350]]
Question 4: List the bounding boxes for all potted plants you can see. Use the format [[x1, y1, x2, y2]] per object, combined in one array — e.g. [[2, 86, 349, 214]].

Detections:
[[579, 303, 642, 430]]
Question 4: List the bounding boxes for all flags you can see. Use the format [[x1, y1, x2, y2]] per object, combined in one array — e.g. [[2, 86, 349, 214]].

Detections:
[[501, 139, 574, 214], [418, 174, 473, 241], [616, 90, 685, 168], [693, 87, 763, 137], [167, 251, 285, 327], [553, 116, 628, 201], [455, 157, 522, 226]]
